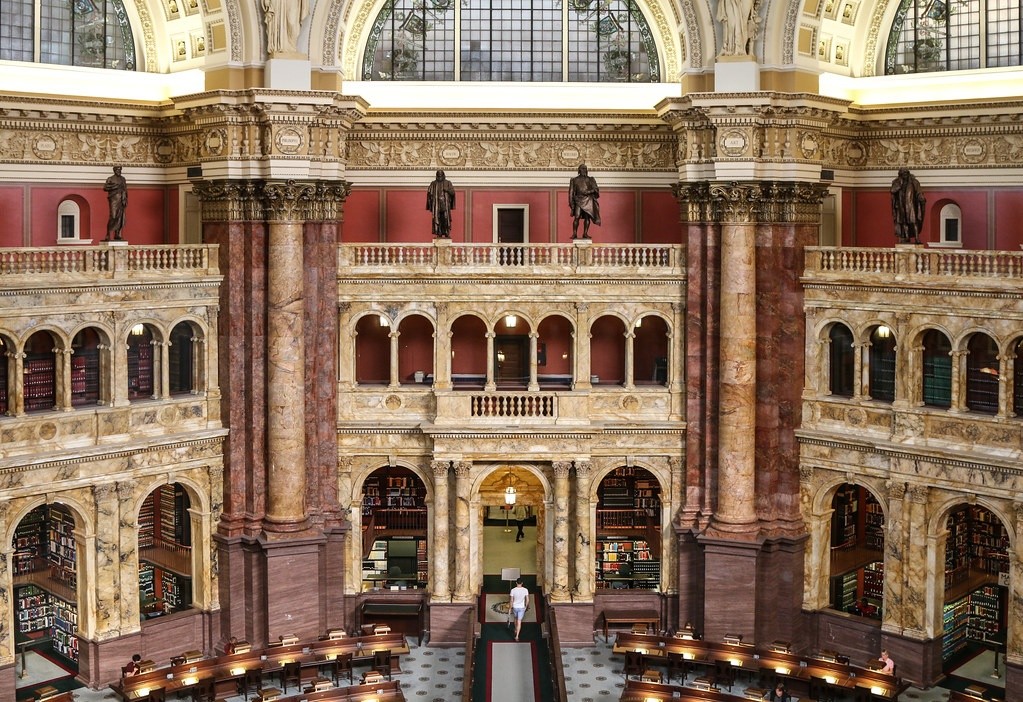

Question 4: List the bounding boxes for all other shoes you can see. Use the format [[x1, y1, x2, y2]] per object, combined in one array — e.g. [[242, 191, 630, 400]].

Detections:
[[521, 533, 524, 538], [515, 637, 520, 642], [516, 540, 521, 542]]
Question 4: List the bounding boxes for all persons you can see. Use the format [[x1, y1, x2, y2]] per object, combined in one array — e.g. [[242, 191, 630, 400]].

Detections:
[[508, 578, 529, 642], [890, 166, 926, 244], [876, 648, 894, 677], [426, 169, 456, 238], [123, 654, 141, 679], [511, 504, 529, 542], [224, 637, 238, 656], [854, 599, 875, 617], [568, 164, 601, 239], [103, 165, 129, 241], [764, 683, 791, 702]]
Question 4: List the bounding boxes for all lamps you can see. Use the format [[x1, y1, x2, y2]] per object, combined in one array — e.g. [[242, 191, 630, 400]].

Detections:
[[980, 367, 999, 375], [132, 323, 143, 335], [497, 353, 505, 362], [506, 314, 517, 328], [504, 467, 515, 504], [878, 325, 890, 339], [965, 684, 989, 700], [33, 685, 58, 701], [562, 352, 569, 360]]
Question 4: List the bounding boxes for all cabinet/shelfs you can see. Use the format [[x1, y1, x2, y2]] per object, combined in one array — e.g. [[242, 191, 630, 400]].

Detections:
[[0, 327, 197, 670], [361, 465, 428, 589], [360, 599, 424, 647], [831, 323, 1010, 665], [594, 465, 662, 591]]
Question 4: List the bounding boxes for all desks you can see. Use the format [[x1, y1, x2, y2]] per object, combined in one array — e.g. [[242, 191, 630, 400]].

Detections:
[[603, 573, 655, 588], [37, 690, 76, 702], [602, 610, 660, 644], [366, 574, 417, 589], [947, 689, 991, 702]]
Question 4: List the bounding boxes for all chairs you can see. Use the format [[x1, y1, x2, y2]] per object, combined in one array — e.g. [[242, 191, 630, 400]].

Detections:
[[612, 623, 912, 702], [18, 696, 35, 702], [108, 621, 410, 702]]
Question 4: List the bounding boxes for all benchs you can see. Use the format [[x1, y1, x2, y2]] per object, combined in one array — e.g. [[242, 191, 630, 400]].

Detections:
[[426, 373, 487, 384], [538, 373, 574, 384]]
